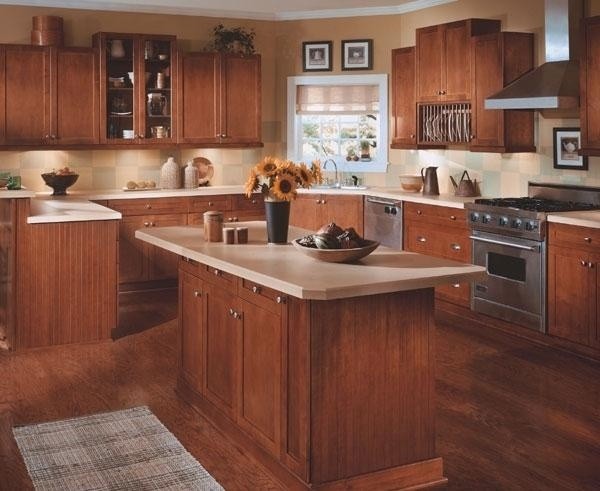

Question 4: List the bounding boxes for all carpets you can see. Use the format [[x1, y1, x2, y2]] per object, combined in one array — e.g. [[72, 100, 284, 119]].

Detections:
[[13, 406, 225, 490]]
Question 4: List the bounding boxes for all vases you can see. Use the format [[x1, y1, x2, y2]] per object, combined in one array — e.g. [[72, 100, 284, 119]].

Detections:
[[266, 201, 290, 245]]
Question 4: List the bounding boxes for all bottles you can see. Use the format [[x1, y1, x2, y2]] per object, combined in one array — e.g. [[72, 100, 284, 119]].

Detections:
[[182, 161, 200, 191], [157, 72, 166, 89], [159, 157, 181, 190]]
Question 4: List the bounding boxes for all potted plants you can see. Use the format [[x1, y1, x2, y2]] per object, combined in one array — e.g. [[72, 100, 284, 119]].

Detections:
[[204, 24, 257, 53]]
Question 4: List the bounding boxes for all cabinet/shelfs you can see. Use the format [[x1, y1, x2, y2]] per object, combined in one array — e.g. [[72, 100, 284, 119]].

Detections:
[[92, 31, 179, 147], [417, 16, 501, 102], [390, 47, 420, 148], [580, 15, 600, 157], [178, 256, 202, 400], [290, 192, 365, 239], [187, 197, 268, 223], [1, 44, 100, 146], [202, 264, 310, 488], [546, 219, 600, 351], [179, 50, 263, 147], [420, 102, 471, 149], [403, 201, 471, 309], [472, 31, 536, 154], [109, 197, 187, 288]]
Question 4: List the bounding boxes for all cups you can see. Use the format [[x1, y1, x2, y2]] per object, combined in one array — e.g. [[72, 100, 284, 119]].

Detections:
[[222, 228, 235, 245], [8, 168, 21, 190], [236, 226, 248, 246]]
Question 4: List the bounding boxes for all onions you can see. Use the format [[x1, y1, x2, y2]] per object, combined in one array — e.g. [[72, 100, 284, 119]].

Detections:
[[298, 222, 361, 249]]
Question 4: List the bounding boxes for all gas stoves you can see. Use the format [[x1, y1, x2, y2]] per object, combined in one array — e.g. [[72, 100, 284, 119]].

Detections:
[[470, 195, 599, 242]]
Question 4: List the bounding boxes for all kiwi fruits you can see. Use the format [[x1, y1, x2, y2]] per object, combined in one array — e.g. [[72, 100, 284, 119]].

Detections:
[[136, 180, 146, 188], [55, 166, 77, 177], [126, 180, 137, 190], [145, 181, 157, 188]]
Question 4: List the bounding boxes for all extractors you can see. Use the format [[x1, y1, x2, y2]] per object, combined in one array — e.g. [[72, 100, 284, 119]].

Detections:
[[484, 2, 587, 111]]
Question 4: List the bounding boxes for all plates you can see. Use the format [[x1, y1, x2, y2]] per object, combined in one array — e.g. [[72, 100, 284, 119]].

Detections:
[[109, 112, 132, 116], [192, 157, 214, 184], [122, 130, 134, 140], [122, 187, 161, 191]]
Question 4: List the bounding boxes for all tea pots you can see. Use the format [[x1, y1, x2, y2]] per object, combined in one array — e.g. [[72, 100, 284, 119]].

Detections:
[[420, 166, 440, 197], [450, 171, 479, 198], [150, 125, 171, 140], [105, 39, 126, 58], [146, 93, 167, 118], [202, 210, 225, 242]]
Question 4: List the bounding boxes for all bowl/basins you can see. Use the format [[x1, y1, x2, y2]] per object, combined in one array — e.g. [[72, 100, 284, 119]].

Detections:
[[294, 237, 380, 266], [0, 178, 10, 189], [42, 173, 80, 196], [399, 176, 423, 192], [157, 54, 168, 62]]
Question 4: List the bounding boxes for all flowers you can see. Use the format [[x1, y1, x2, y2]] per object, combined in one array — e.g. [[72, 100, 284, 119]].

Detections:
[[244, 157, 313, 201]]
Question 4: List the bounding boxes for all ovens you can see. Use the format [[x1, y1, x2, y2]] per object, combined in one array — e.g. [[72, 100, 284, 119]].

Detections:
[[468, 229, 546, 334]]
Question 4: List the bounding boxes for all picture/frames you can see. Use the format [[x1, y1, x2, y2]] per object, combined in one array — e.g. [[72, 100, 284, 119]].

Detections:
[[552, 126, 589, 170], [302, 40, 333, 71], [342, 38, 372, 69]]
